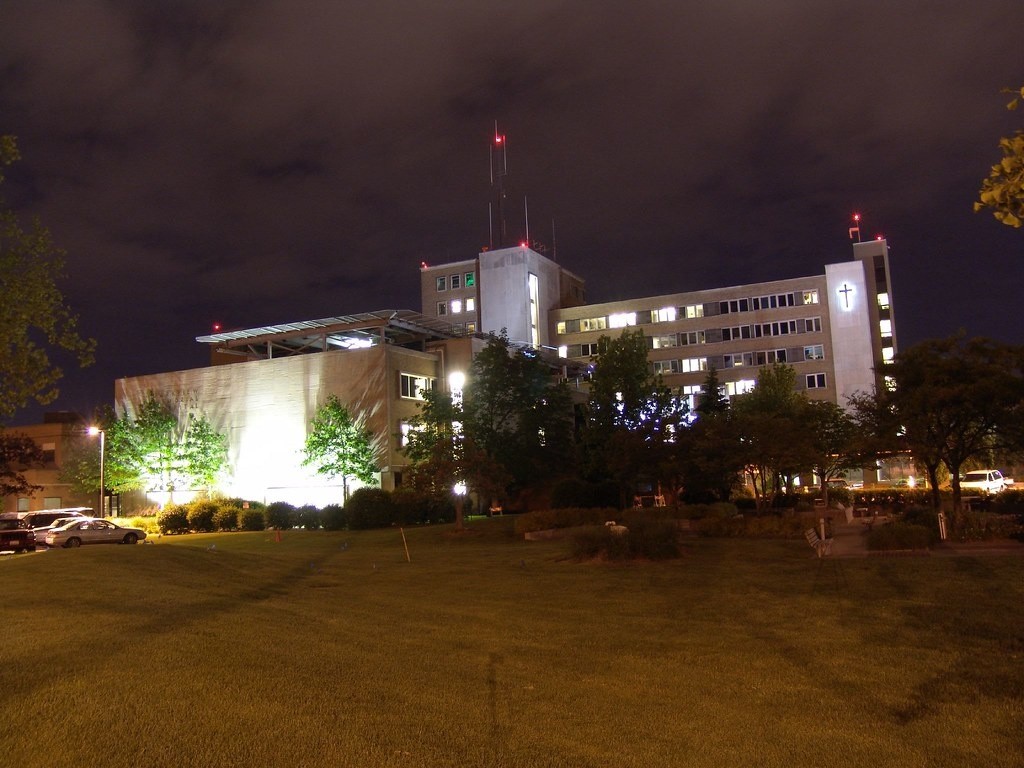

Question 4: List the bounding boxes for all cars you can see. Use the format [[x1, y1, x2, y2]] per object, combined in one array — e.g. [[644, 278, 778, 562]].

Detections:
[[46, 518, 148, 548], [32, 517, 82, 543], [819, 479, 851, 491], [959, 470, 1004, 497], [0, 518, 36, 552]]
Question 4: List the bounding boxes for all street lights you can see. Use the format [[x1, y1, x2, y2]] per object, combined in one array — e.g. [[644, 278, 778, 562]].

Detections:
[[89, 426, 106, 518], [448, 369, 466, 530]]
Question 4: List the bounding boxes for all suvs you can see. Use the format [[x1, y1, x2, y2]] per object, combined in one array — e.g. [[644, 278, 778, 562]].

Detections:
[[24, 512, 84, 529]]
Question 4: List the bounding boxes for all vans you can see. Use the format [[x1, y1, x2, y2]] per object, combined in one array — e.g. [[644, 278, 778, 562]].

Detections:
[[45, 508, 98, 517]]
